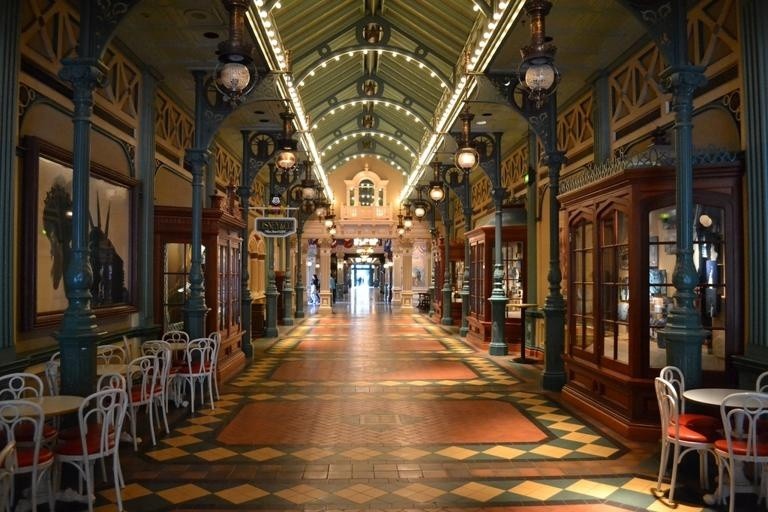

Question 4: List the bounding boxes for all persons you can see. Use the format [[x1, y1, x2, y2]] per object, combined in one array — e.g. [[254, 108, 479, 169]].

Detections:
[[310, 279, 318, 304], [309, 274, 320, 304]]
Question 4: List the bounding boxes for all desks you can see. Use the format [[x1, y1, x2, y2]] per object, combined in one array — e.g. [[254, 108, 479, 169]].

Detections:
[[2, 396, 89, 510]]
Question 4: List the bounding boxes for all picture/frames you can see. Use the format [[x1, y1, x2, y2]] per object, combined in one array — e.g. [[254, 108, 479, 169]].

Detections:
[[18, 138, 141, 335]]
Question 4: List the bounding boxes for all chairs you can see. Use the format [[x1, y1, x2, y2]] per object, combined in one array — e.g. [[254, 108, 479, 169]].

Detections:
[[652, 363, 768, 511], [53, 389, 128, 511], [3, 400, 55, 511], [47, 328, 222, 450], [1, 374, 57, 449]]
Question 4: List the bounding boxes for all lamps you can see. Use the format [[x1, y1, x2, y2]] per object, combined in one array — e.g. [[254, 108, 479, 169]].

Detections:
[[300, 159, 337, 238], [514, 6, 562, 108], [396, 163, 446, 240], [455, 109, 479, 177], [275, 110, 301, 173], [212, 0, 258, 105]]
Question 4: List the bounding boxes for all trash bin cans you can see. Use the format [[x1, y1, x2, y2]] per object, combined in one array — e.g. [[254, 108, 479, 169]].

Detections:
[[252, 304, 265, 338]]
